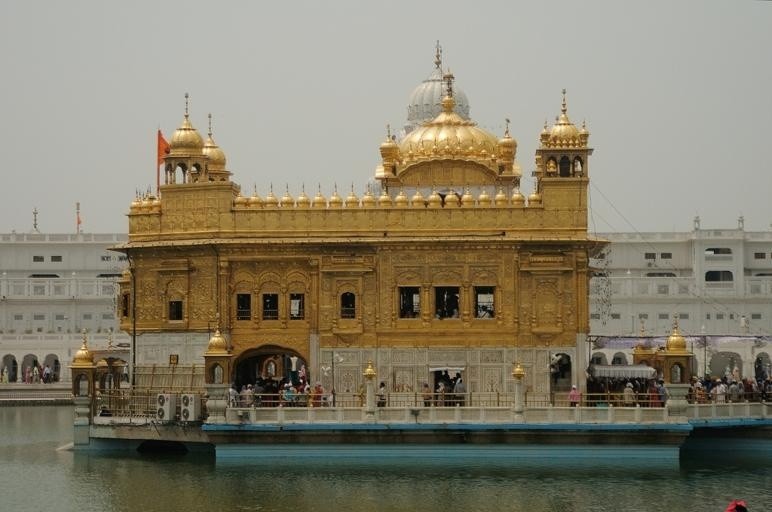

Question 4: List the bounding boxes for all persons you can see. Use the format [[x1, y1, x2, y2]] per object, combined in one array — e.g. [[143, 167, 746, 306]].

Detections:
[[375, 382, 388, 406], [566, 375, 669, 407], [402, 300, 494, 319], [230, 364, 339, 406], [421, 369, 466, 406], [122, 363, 131, 383], [686, 372, 771, 402], [1, 364, 55, 384]]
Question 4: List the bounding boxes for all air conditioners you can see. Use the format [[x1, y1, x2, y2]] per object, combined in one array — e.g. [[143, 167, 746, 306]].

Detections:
[[156, 393, 202, 422]]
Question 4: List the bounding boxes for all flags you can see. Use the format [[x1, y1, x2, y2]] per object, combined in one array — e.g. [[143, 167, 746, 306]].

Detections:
[[157, 131, 171, 167]]
